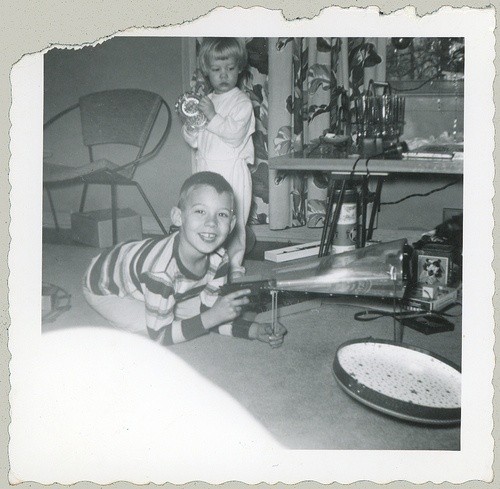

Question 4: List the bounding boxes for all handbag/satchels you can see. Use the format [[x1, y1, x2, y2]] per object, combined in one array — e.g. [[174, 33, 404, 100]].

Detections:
[[354, 300, 462, 334]]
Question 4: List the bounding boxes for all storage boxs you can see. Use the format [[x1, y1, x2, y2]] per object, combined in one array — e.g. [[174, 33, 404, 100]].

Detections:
[[71, 207, 142, 250]]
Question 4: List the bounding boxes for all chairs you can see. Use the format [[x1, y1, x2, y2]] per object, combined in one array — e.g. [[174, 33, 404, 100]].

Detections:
[[44, 88, 172, 246]]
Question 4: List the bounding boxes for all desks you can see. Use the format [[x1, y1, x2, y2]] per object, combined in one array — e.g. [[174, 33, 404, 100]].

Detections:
[[268, 150, 463, 257]]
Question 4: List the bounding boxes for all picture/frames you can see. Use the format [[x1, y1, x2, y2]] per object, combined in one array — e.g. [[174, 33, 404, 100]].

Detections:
[[413, 249, 451, 287], [374, 37, 464, 101]]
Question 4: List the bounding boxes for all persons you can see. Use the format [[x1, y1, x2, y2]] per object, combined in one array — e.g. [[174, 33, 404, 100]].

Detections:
[[181, 38, 256, 281], [83, 171, 287, 348]]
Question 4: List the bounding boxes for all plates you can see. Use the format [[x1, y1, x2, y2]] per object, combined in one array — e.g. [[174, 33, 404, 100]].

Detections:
[[333, 336, 460, 426]]
